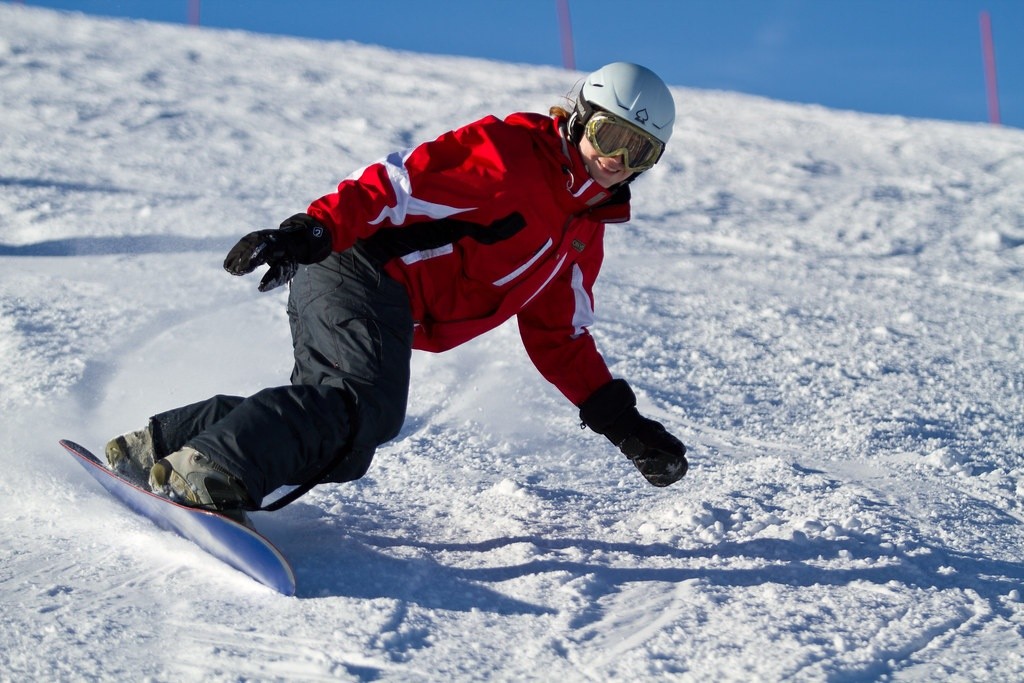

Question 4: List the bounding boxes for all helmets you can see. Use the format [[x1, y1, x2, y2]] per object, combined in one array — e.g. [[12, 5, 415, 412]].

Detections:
[[575, 62, 675, 164]]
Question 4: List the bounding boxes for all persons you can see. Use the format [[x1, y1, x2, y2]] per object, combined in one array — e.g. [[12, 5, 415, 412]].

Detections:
[[106, 61, 690, 531]]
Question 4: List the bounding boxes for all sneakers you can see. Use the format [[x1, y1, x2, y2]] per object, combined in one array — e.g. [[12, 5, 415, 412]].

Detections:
[[105, 426, 154, 492], [149, 446, 256, 533]]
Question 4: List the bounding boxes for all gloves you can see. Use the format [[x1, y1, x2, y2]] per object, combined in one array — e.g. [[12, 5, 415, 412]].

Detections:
[[223, 214, 333, 293], [578, 378, 688, 487]]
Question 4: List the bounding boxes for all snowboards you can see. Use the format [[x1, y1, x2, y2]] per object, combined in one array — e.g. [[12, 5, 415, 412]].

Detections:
[[58, 437, 297, 599]]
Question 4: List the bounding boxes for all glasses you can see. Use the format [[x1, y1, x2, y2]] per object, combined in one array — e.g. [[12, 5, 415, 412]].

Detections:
[[585, 111, 663, 172]]
